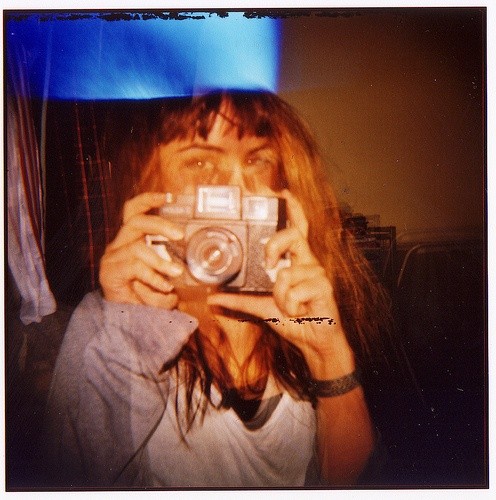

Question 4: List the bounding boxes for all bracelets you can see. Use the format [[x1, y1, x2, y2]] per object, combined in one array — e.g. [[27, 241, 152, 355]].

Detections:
[[302, 368, 362, 398]]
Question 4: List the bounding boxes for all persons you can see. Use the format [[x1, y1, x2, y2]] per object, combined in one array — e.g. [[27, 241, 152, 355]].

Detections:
[[39, 89, 431, 487]]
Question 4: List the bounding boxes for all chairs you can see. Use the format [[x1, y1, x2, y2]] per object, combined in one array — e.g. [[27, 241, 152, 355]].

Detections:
[[383, 242, 430, 412]]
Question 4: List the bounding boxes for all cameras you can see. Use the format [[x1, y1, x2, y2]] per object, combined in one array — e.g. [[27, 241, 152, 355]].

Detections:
[[146, 185, 285, 294]]
